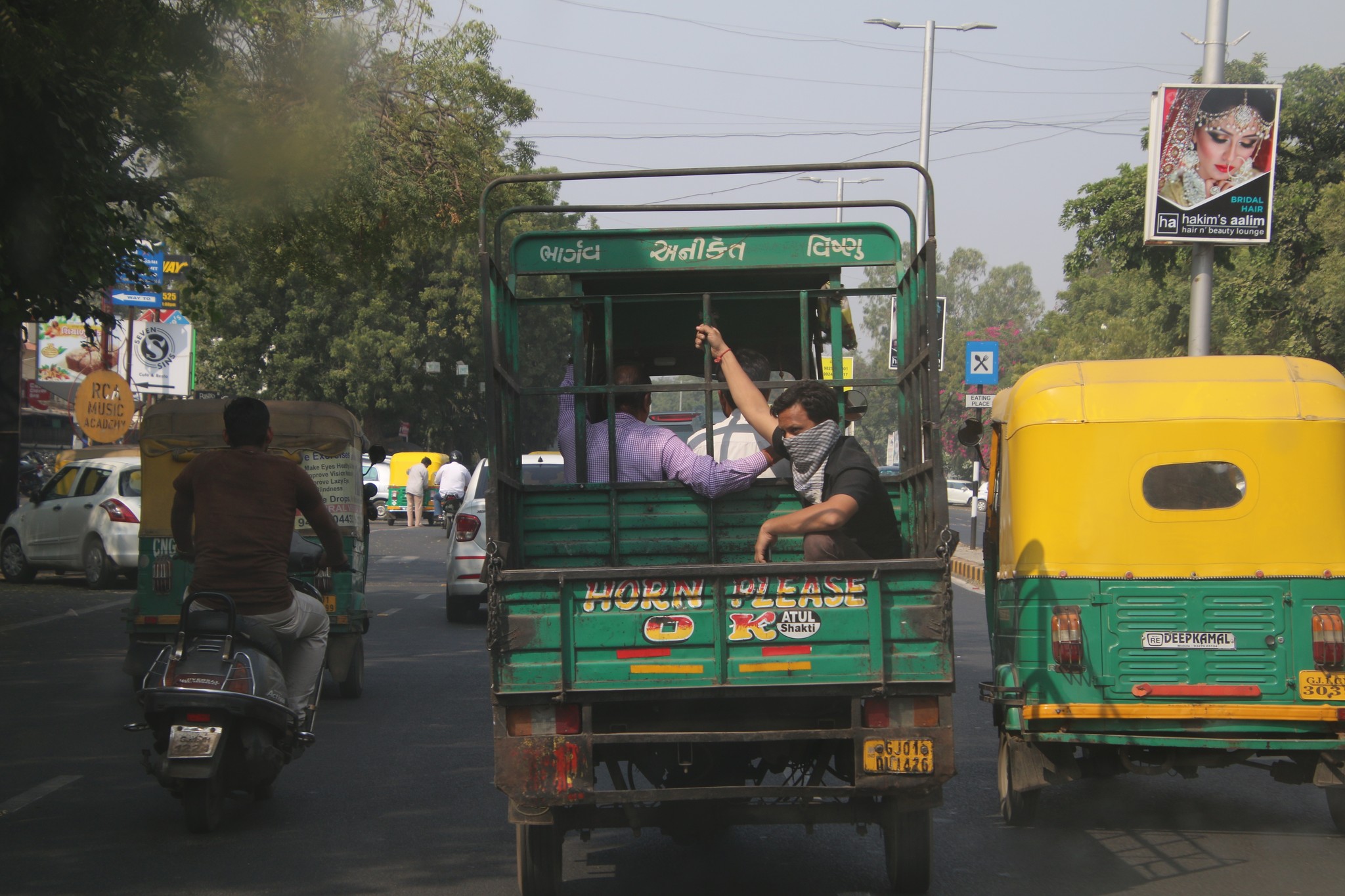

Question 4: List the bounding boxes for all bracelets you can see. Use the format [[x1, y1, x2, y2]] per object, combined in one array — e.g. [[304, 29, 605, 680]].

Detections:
[[175, 543, 195, 555], [713, 349, 731, 363]]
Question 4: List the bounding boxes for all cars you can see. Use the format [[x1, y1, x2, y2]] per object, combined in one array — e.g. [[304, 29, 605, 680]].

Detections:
[[946, 479, 999, 512], [0, 457, 142, 590], [361, 454, 392, 521], [442, 455, 564, 622]]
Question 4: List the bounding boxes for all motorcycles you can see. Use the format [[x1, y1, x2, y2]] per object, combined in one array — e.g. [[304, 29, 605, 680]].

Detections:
[[129, 399, 386, 698], [434, 471, 467, 539], [17, 441, 141, 499], [386, 452, 448, 526], [470, 156, 959, 895], [958, 356, 1345, 824]]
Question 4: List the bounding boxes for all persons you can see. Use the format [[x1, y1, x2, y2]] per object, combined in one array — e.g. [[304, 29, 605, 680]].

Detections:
[[433, 450, 471, 519], [169, 396, 349, 730], [686, 349, 794, 480], [695, 323, 903, 562], [557, 311, 785, 498], [405, 457, 431, 528], [1161, 88, 1276, 209]]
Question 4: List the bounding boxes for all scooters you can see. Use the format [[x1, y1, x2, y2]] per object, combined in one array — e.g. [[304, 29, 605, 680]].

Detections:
[[117, 530, 356, 832]]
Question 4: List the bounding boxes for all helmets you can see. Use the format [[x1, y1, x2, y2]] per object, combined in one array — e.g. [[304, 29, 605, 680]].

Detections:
[[449, 450, 463, 463]]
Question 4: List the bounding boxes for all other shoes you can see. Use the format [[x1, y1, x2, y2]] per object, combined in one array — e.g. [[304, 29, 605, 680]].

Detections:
[[434, 517, 441, 521]]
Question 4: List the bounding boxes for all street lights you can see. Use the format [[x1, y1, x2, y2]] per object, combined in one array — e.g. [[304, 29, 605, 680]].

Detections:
[[863, 17, 997, 258], [798, 176, 883, 226]]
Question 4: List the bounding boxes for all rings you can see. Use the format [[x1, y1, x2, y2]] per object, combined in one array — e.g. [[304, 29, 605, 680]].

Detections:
[[1210, 186, 1220, 195]]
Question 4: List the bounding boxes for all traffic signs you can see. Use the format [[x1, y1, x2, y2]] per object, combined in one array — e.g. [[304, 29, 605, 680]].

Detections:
[[112, 289, 162, 308], [117, 320, 191, 395]]
